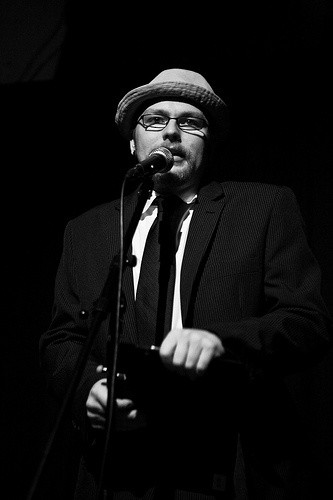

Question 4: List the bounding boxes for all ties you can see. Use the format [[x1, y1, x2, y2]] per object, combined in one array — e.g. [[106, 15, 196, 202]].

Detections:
[[132, 194, 186, 374]]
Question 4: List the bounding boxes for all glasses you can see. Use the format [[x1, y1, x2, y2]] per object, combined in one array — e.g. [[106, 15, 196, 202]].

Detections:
[[142, 113, 205, 131]]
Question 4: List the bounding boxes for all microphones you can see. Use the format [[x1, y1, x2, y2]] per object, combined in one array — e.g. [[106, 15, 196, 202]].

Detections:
[[128, 146, 174, 177]]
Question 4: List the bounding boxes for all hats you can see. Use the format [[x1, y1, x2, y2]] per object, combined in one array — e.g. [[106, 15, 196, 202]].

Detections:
[[114, 69, 226, 125]]
